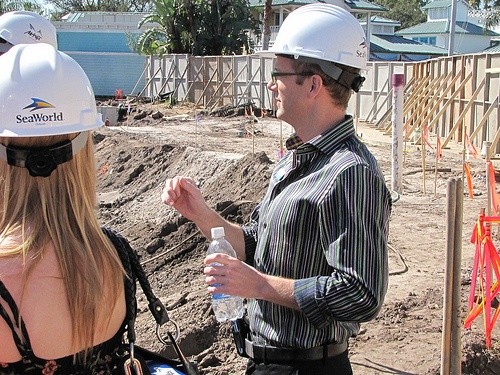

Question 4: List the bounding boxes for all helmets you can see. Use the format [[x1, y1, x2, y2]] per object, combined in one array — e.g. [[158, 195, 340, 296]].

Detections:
[[0, 12, 58, 53], [254, 2, 369, 71], [0, 42, 104, 139]]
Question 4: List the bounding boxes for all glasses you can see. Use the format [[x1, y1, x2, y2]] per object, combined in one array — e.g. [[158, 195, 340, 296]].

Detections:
[[270, 70, 330, 86]]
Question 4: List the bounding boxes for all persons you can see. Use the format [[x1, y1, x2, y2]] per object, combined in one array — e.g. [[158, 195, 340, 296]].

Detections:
[[0, 12, 59, 55], [0, 42, 130, 375], [159, 2, 393, 375]]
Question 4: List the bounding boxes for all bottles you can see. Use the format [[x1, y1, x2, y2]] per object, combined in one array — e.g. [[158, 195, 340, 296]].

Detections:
[[205, 227, 244, 323]]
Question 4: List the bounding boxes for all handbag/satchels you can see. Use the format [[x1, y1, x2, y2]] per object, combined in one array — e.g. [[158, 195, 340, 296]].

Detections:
[[100, 225, 198, 374]]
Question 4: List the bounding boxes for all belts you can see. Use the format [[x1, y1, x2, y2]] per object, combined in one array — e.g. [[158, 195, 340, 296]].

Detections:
[[241, 337, 349, 364]]
